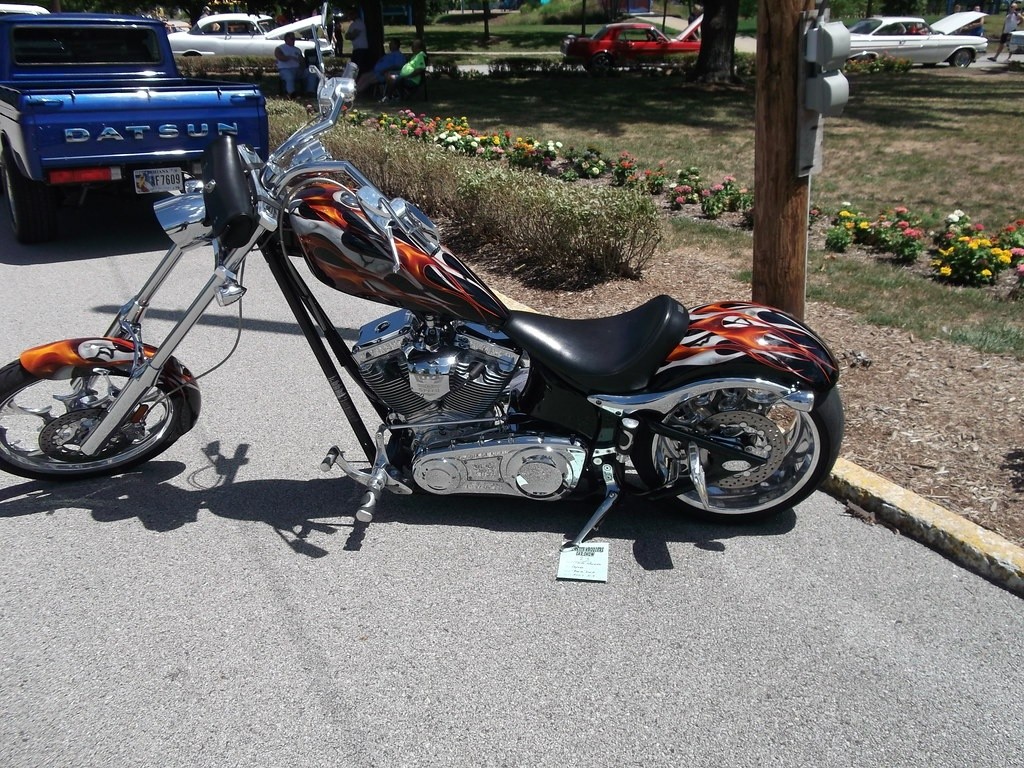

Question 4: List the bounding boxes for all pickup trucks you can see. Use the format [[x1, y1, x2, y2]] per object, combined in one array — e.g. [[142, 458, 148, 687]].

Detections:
[[0, 13, 270, 243]]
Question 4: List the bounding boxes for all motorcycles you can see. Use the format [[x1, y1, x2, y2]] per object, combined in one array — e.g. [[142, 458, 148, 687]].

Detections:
[[1, 61, 846, 554]]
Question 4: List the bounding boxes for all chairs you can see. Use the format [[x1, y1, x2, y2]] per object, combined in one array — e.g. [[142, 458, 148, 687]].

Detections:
[[374, 70, 429, 104]]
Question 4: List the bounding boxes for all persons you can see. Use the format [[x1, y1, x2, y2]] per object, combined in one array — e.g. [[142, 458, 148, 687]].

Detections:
[[274, 32, 317, 98], [332, 18, 345, 56], [199, 6, 212, 19], [356, 38, 407, 93], [211, 23, 220, 33], [987, 3, 1024, 63], [688, 4, 703, 40], [379, 40, 427, 103], [344, 6, 368, 64], [951, 4, 961, 14], [968, 6, 984, 36]]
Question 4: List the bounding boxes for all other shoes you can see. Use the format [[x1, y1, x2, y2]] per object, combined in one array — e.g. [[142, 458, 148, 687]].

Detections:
[[1004, 60, 1011, 63], [376, 96, 390, 105], [392, 96, 401, 106], [987, 57, 996, 62]]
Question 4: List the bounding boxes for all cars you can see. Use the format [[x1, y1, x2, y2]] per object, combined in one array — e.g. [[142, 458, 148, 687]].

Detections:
[[847, 11, 990, 67], [1007, 31, 1024, 54], [0, 4, 50, 15], [168, 2, 338, 57], [560, 14, 703, 70]]
